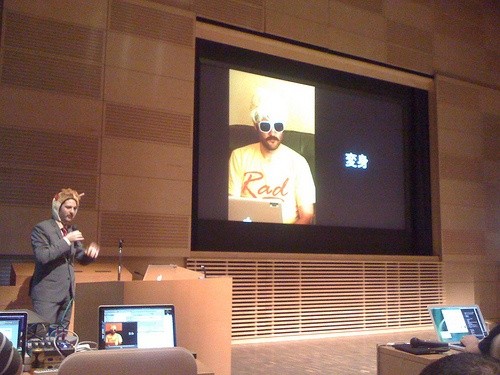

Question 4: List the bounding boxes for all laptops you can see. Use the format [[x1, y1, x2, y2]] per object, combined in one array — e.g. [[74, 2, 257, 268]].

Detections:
[[0, 310, 29, 374], [426, 304, 489, 351]]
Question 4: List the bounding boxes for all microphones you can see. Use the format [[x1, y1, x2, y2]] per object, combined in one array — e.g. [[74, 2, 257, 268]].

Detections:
[[410, 337, 449, 348], [72, 224, 79, 248]]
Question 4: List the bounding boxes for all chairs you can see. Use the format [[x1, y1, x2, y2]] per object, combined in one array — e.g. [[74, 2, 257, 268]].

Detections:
[[59, 347, 197, 375]]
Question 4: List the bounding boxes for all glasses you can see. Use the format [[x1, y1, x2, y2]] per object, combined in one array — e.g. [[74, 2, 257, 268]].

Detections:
[[258, 121, 284, 133]]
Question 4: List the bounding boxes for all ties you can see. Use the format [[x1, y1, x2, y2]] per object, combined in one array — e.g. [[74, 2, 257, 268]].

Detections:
[[61, 227, 68, 236]]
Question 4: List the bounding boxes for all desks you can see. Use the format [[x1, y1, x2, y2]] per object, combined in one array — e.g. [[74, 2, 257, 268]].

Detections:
[[377, 342, 463, 375]]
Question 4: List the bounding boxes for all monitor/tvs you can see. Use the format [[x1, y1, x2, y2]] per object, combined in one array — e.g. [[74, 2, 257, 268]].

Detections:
[[97, 304, 177, 350]]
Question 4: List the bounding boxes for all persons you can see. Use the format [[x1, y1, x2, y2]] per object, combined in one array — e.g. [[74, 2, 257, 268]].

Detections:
[[28, 189, 99, 335], [0, 332, 22, 375], [460, 325, 500, 363], [106, 325, 122, 346], [228, 92, 316, 223]]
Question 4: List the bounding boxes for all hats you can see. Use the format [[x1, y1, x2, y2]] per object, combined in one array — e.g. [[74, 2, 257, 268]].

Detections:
[[52, 189, 84, 224]]
